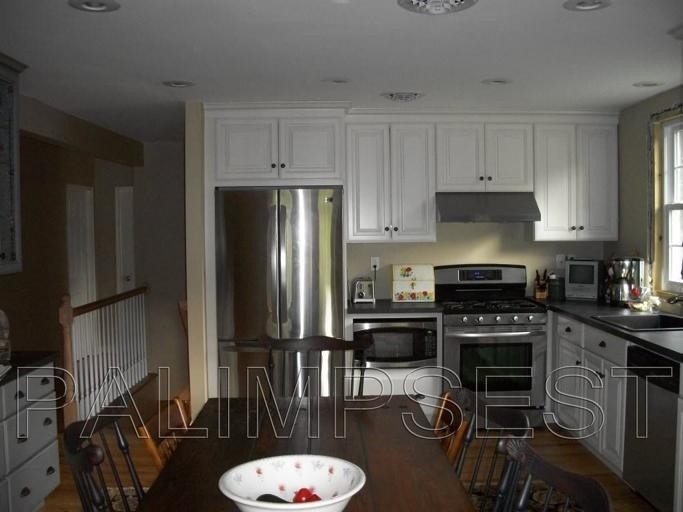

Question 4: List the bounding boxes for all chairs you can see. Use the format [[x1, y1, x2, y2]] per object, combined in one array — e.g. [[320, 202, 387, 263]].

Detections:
[[430, 392, 476, 477], [456, 404, 531, 512], [493, 438, 612, 512], [259, 333, 375, 397], [63, 415, 144, 512], [138, 396, 192, 473]]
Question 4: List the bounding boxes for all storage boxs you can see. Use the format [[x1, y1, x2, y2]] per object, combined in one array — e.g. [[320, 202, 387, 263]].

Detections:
[[394, 264, 435, 304]]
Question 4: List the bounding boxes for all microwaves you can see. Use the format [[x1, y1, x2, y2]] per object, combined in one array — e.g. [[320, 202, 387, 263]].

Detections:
[[352, 318, 437, 367]]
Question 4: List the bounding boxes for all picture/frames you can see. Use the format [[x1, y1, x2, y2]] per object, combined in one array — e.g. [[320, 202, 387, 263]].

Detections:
[[0, 67, 25, 276]]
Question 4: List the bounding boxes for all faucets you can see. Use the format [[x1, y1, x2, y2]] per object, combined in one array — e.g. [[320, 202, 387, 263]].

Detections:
[[668, 294, 683, 305]]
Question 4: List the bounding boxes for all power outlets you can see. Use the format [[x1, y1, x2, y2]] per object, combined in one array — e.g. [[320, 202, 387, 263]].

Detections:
[[371, 256, 379, 271]]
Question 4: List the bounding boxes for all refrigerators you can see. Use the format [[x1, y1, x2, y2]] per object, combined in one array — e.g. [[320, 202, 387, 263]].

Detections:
[[214, 185, 343, 398]]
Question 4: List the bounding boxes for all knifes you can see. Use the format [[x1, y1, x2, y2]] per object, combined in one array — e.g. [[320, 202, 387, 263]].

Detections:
[[535, 269, 549, 286]]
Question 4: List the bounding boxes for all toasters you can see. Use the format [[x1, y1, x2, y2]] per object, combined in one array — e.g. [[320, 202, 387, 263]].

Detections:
[[351, 275, 377, 305]]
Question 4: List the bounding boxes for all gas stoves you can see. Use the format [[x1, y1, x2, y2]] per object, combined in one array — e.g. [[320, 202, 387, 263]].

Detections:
[[435, 296, 548, 314]]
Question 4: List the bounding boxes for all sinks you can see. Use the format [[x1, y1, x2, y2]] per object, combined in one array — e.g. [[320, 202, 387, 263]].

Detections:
[[590, 312, 683, 333]]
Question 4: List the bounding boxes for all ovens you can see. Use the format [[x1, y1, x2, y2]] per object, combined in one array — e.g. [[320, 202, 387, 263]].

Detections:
[[443, 324, 547, 408]]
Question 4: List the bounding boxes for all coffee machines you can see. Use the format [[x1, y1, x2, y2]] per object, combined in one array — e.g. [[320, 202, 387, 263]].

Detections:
[[610, 257, 644, 308]]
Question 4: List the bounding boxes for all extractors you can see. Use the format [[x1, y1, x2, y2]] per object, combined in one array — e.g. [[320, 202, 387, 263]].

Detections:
[[435, 191, 541, 223]]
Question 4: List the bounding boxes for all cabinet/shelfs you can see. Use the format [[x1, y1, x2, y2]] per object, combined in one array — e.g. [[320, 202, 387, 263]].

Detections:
[[434, 113, 534, 194], [545, 307, 626, 479], [0, 351, 61, 512], [204, 101, 345, 187], [534, 111, 619, 243], [343, 113, 438, 244]]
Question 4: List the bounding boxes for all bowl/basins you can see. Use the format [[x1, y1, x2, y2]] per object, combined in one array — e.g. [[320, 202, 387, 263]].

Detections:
[[216, 453, 367, 512]]
